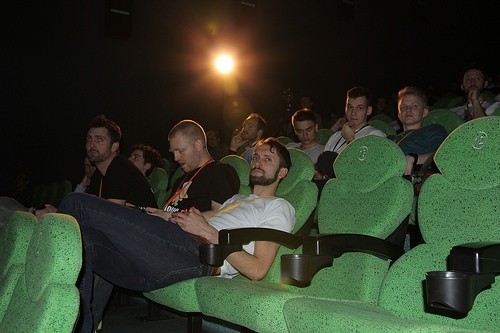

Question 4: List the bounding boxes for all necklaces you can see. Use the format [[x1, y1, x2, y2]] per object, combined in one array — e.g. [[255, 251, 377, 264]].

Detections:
[[162, 158, 212, 211], [100, 176, 104, 199], [208, 196, 267, 221]]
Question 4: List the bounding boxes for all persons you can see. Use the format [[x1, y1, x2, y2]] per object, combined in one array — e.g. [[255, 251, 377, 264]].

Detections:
[[7, 171, 38, 211], [126, 144, 162, 179], [224, 112, 271, 160], [35, 117, 158, 238], [283, 63, 495, 196], [69, 154, 99, 194], [57, 138, 298, 333], [89, 119, 242, 330]]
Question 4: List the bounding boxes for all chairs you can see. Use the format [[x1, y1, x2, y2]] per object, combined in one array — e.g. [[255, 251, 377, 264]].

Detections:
[[1, 100, 500, 333]]
